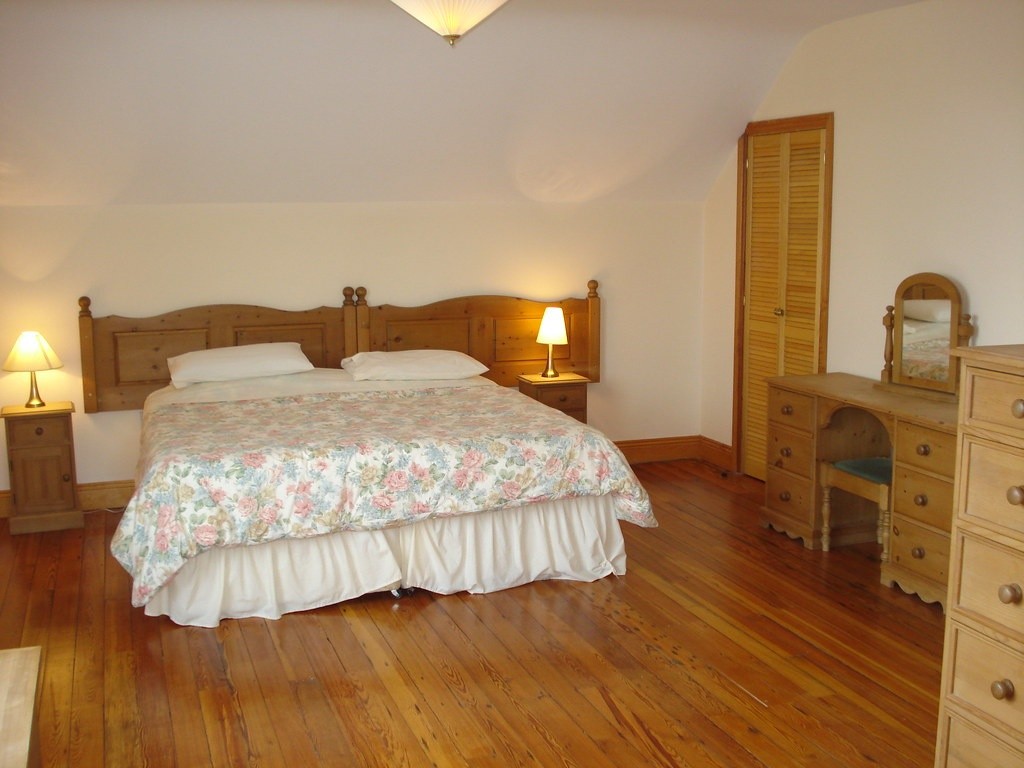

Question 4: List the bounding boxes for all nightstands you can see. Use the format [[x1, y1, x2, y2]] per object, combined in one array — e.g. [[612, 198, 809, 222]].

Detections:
[[515, 372, 591, 424], [80, 279, 659, 629], [0, 401, 84, 535]]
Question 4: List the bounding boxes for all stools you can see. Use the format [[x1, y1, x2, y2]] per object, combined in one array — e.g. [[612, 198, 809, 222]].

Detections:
[[819, 458, 893, 567]]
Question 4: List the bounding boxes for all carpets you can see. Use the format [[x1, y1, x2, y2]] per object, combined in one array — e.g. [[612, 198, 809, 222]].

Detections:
[[0, 645, 42, 768]]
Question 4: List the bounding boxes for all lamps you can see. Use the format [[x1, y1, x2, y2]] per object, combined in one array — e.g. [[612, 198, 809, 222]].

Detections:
[[390, 0, 509, 47], [1, 331, 64, 408], [534, 306, 569, 378]]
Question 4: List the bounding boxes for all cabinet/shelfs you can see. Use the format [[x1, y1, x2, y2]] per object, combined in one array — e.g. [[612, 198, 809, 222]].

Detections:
[[934, 345, 1024, 767], [759, 372, 958, 615]]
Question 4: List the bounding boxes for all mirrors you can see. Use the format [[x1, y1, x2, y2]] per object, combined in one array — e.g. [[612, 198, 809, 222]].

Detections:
[[890, 272, 962, 393]]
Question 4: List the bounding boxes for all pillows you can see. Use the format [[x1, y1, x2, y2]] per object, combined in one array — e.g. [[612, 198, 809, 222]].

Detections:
[[166, 343, 315, 389], [340, 349, 490, 383]]
